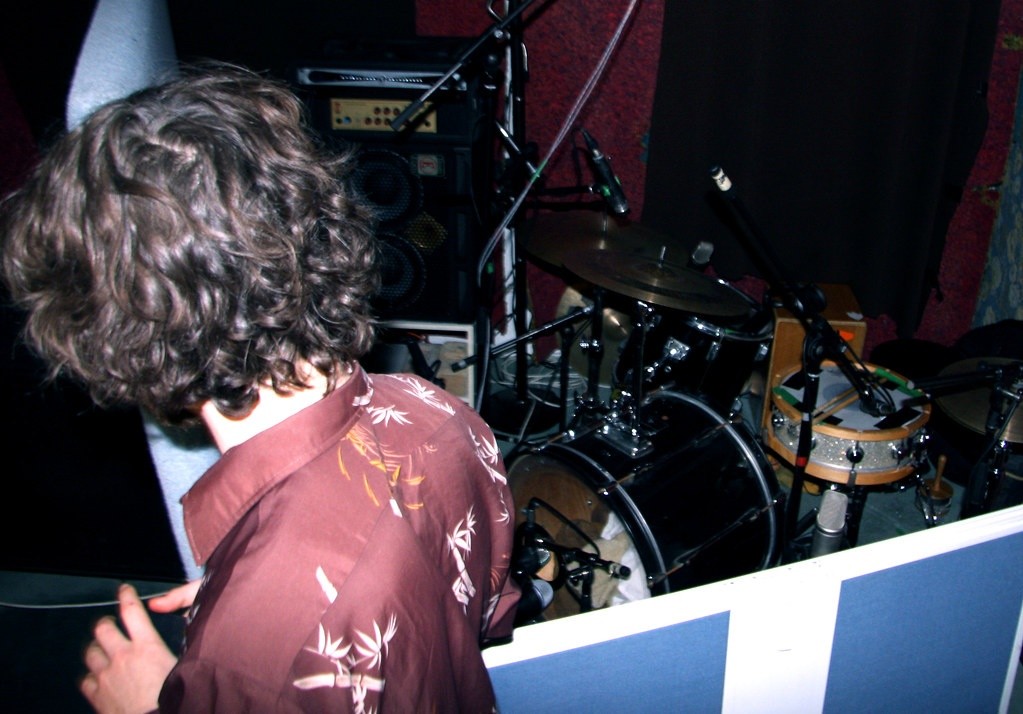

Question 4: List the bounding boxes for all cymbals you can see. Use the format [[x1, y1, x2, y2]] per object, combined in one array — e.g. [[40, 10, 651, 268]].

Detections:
[[563, 256, 750, 317], [514, 207, 689, 274], [928, 320, 1023, 443]]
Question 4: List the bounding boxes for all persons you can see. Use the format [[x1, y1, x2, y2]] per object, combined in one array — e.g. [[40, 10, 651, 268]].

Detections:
[[0, 62, 523, 714]]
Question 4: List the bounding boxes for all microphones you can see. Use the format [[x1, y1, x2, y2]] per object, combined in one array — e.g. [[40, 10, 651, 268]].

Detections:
[[493, 120, 541, 179], [512, 579, 554, 628], [860, 400, 897, 417], [579, 128, 629, 213], [520, 501, 542, 576]]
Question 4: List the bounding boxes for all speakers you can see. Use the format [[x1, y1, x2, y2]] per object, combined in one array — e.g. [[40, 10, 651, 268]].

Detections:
[[305, 132, 496, 332]]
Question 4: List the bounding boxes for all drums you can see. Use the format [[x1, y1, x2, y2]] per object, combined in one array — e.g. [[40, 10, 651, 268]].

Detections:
[[507, 389, 778, 621], [614, 287, 772, 408], [760, 358, 931, 487]]
